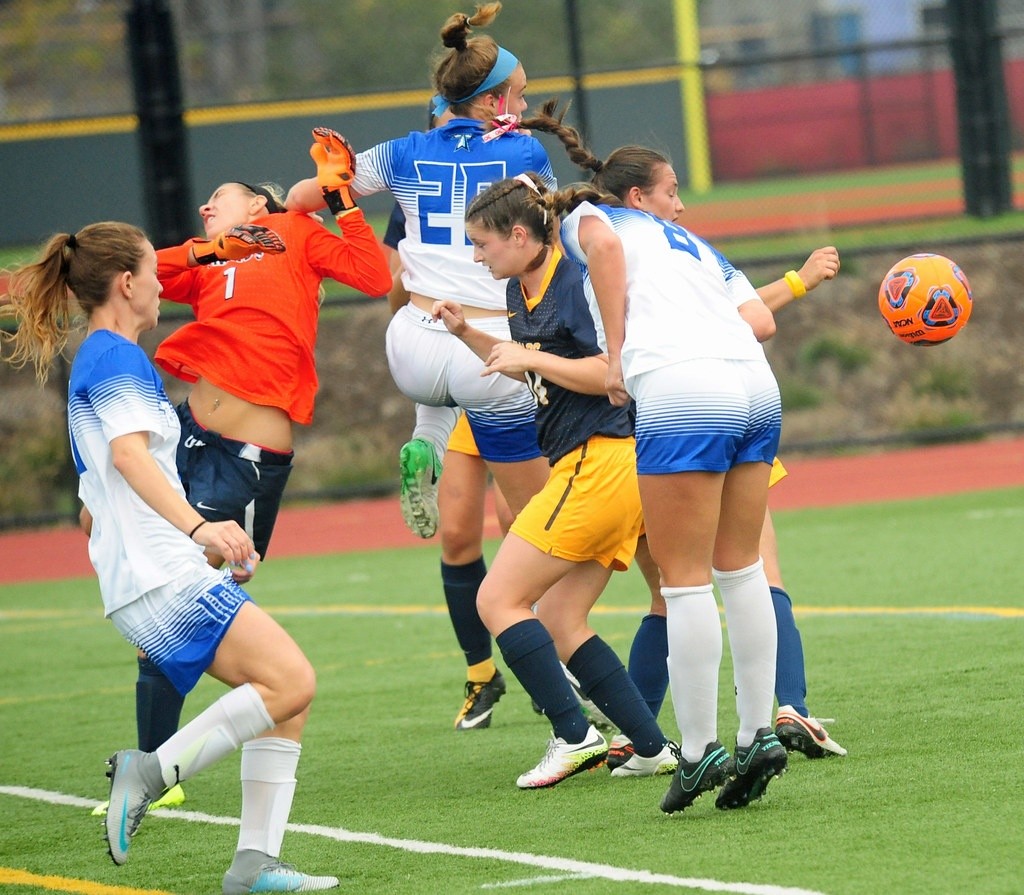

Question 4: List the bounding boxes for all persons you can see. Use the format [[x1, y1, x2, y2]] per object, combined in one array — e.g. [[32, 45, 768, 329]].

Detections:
[[1, 220, 340, 893], [557, 182, 788, 811], [384, 91, 609, 729], [92, 123, 393, 811], [511, 99, 848, 760], [349, 1, 612, 734], [430, 169, 681, 789]]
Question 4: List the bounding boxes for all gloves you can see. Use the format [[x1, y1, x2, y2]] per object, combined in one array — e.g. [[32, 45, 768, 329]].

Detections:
[[310, 128, 356, 214], [191, 225, 286, 263]]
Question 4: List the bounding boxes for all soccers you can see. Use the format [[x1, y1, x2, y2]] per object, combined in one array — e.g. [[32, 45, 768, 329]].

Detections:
[[878, 253, 973, 347]]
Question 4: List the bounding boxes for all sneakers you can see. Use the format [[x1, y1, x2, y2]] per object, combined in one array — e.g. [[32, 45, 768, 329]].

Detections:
[[92, 783, 184, 815], [516, 723, 608, 789], [660, 738, 734, 814], [105, 749, 152, 866], [222, 861, 339, 895], [774, 704, 847, 758], [399, 438, 441, 539], [610, 741, 680, 777], [606, 735, 633, 767], [560, 662, 616, 734], [455, 668, 507, 730], [715, 726, 786, 809]]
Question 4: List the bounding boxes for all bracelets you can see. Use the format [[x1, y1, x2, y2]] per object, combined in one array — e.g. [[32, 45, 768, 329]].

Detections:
[[784, 270, 806, 299], [190, 520, 207, 538]]
